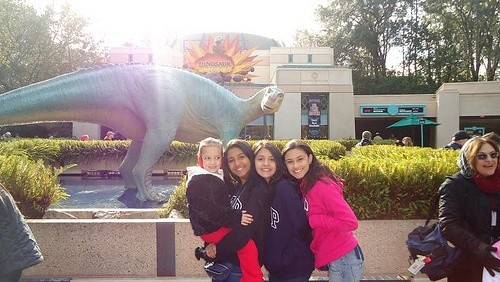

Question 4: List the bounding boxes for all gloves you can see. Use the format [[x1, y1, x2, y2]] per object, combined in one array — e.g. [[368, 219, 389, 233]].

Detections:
[[478, 243, 500, 277]]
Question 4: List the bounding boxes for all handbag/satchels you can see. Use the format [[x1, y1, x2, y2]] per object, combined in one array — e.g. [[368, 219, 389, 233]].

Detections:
[[405, 221, 464, 281], [204, 261, 235, 281]]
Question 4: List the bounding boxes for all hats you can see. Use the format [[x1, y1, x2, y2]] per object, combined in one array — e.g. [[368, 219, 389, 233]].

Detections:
[[452, 130, 473, 141]]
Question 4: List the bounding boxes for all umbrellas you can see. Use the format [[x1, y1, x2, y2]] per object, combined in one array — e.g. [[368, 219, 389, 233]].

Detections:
[[385, 114, 441, 143]]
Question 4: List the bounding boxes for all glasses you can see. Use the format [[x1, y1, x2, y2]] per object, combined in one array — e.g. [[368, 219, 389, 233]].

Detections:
[[477, 151, 498, 160]]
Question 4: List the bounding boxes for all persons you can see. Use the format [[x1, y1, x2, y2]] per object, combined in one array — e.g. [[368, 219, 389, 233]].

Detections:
[[185, 137, 265, 282], [280, 139, 364, 282], [358, 131, 500, 149], [439, 137, 500, 282], [1, 131, 127, 142], [251, 140, 316, 282], [205, 139, 271, 282], [0, 182, 44, 282]]
